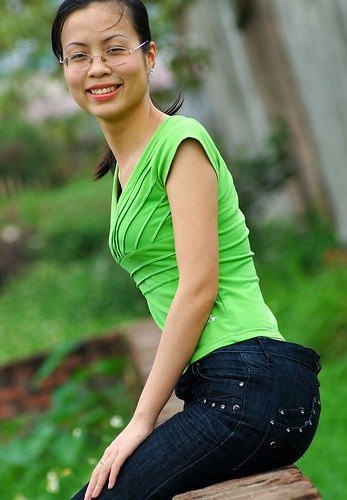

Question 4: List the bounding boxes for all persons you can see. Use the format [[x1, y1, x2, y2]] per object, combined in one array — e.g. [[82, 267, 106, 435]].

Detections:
[[51, 0, 323, 500]]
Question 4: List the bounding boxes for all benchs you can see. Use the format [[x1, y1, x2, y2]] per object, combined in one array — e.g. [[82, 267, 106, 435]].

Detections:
[[122, 316, 326, 500]]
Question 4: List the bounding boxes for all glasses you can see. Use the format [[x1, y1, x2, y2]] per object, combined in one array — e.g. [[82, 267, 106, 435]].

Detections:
[[59, 40, 148, 69]]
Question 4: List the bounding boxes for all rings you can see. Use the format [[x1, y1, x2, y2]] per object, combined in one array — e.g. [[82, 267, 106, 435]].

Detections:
[[99, 460, 105, 465]]
